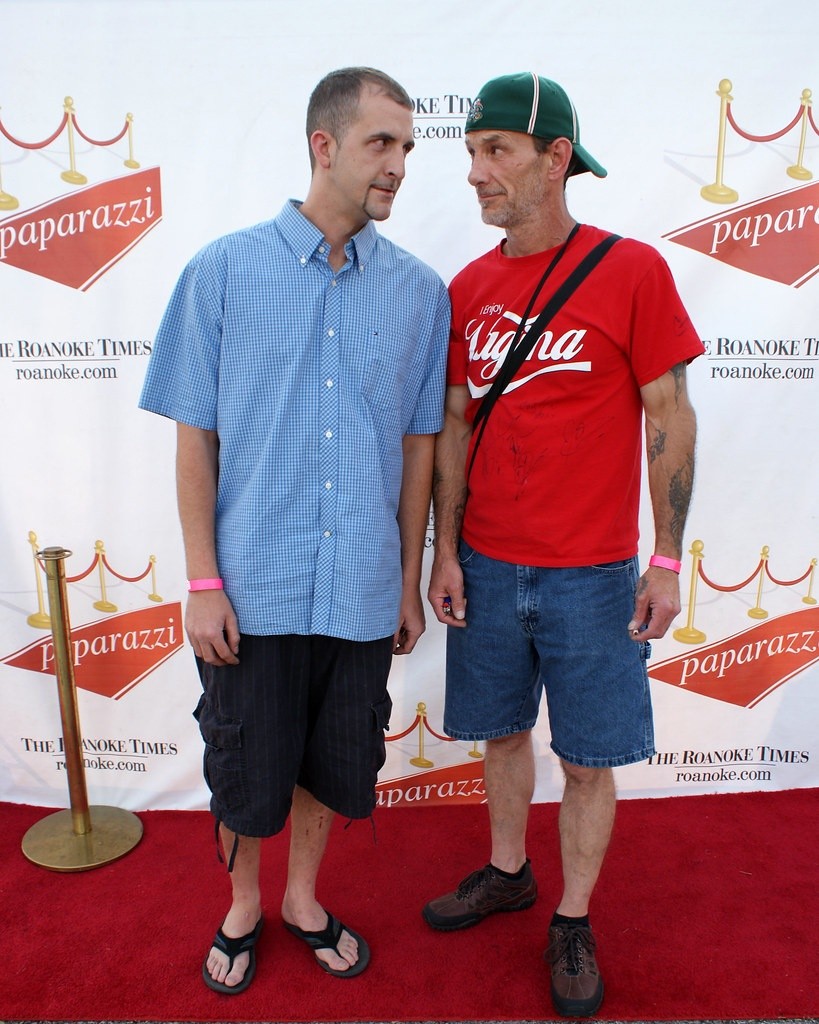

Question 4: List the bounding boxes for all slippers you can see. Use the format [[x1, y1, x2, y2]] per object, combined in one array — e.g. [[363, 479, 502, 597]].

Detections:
[[202, 905, 265, 995], [281, 903, 372, 979]]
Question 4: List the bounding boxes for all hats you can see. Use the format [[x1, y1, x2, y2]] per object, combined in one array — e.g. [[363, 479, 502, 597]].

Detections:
[[464, 70, 608, 183]]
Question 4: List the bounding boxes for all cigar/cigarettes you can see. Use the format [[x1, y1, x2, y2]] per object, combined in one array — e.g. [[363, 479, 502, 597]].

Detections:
[[633, 630, 638, 636]]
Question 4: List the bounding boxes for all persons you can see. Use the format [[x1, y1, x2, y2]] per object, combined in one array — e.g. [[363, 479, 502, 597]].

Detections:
[[138, 70, 450, 994], [423, 75, 706, 1017]]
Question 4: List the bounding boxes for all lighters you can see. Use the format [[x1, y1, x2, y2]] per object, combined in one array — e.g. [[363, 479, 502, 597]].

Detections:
[[443, 598, 450, 615]]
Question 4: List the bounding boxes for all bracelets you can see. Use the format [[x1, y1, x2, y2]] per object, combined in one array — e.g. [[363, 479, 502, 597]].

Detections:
[[186, 579, 223, 592], [649, 555, 681, 574]]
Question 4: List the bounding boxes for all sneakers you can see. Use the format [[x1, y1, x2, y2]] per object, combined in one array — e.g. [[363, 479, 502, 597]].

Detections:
[[422, 860, 539, 933], [547, 921, 605, 1018]]
